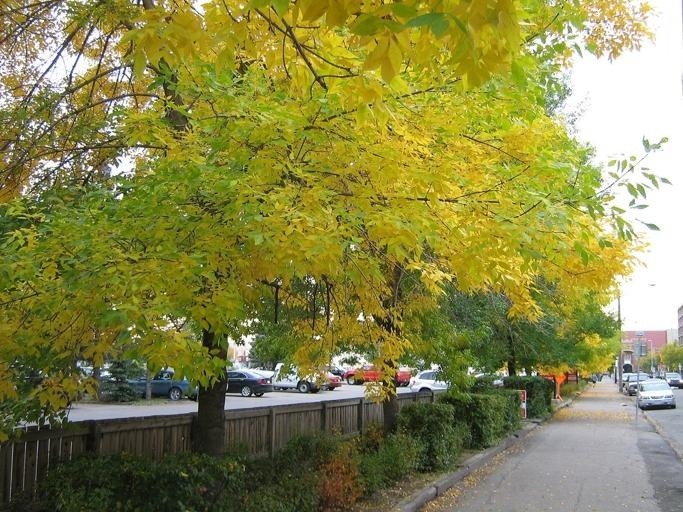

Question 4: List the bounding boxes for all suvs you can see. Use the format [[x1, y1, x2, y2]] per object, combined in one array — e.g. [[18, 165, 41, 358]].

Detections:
[[407, 369, 453, 394]]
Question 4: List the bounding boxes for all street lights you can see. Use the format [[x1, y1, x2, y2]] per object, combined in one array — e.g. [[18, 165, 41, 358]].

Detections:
[[647, 339, 653, 368], [617, 282, 656, 394]]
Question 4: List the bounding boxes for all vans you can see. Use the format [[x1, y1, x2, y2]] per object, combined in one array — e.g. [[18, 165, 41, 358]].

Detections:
[[271, 362, 329, 393]]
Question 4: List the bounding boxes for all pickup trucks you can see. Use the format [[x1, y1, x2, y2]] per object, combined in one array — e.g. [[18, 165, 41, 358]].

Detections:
[[343, 364, 411, 387]]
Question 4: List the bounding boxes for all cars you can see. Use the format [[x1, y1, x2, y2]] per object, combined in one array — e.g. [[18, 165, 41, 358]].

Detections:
[[226, 370, 273, 398], [622, 370, 665, 396], [468, 371, 504, 390], [663, 372, 680, 387], [634, 379, 676, 411], [318, 370, 341, 391], [325, 365, 344, 382], [678, 373, 682, 389], [101, 363, 198, 402]]
